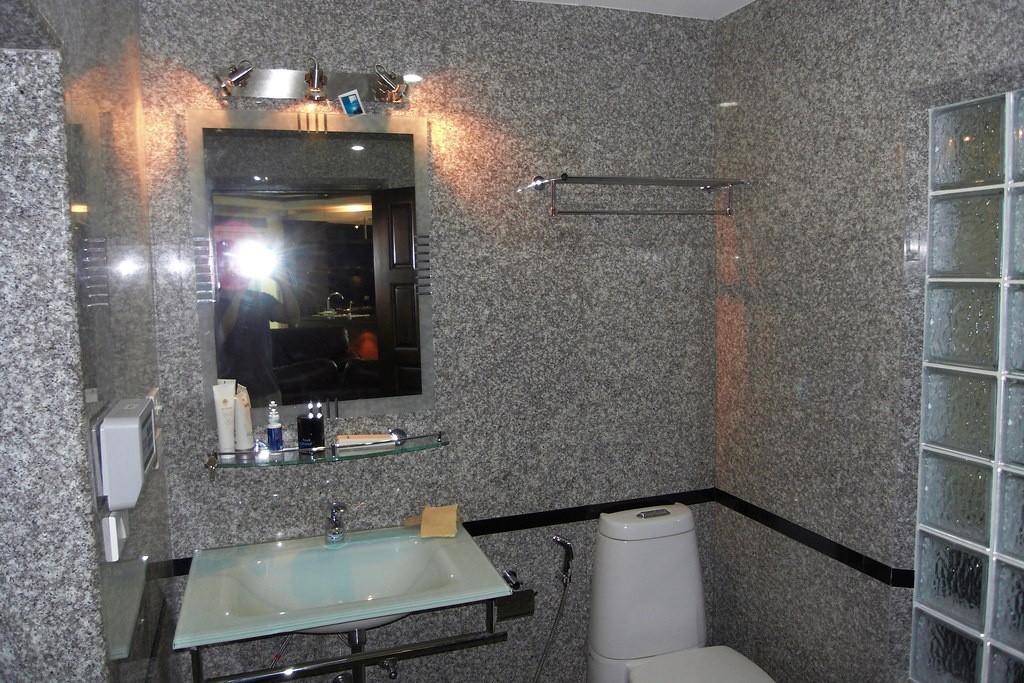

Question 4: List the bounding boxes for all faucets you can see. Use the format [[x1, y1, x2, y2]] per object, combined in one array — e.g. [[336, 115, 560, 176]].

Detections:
[[324, 501, 348, 545]]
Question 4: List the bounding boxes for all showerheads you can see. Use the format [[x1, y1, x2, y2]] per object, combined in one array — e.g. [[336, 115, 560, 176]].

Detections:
[[552, 533, 575, 578]]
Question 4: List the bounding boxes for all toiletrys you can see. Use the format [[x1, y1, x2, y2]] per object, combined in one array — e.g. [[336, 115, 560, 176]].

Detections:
[[217, 379, 236, 385], [233, 391, 255, 450], [236, 383, 252, 409], [266, 408, 284, 451], [296, 400, 325, 456], [212, 381, 236, 460], [316, 399, 323, 414], [264, 400, 280, 416]]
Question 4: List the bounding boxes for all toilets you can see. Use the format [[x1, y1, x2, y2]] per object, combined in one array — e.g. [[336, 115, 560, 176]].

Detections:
[[586, 501, 777, 683]]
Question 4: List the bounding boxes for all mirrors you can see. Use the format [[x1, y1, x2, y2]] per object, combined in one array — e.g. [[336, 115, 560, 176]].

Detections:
[[186, 107, 433, 433]]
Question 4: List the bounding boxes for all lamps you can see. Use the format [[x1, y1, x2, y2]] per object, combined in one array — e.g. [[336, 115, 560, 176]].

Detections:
[[373, 64, 410, 101], [305, 58, 330, 101], [214, 59, 255, 95]]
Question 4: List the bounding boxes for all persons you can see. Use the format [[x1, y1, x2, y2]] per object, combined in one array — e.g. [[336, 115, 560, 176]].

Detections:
[[216, 240, 300, 407]]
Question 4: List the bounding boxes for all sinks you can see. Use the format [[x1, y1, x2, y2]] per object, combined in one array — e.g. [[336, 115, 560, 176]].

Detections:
[[172, 521, 514, 650]]
